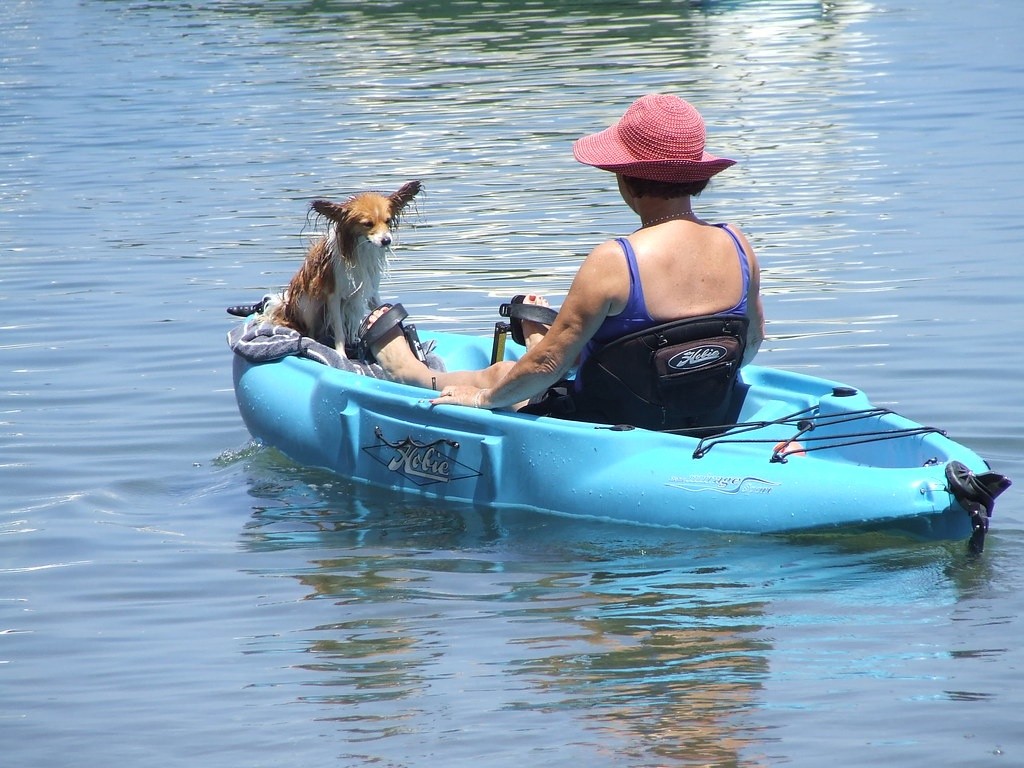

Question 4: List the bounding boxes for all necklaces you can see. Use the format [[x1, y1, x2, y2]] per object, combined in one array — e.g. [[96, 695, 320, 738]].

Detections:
[[642, 211, 694, 227]]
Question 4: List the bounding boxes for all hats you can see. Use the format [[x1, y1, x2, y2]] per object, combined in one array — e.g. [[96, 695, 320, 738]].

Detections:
[[573, 93, 737, 183]]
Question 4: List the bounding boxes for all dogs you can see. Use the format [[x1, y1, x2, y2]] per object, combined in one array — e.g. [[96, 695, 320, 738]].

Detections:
[[252, 180, 426, 361]]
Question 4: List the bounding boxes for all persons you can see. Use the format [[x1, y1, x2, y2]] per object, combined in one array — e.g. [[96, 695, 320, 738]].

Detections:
[[367, 95, 763, 437]]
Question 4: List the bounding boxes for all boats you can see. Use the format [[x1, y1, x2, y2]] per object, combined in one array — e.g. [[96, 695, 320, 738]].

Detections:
[[223, 312, 1012, 561]]
